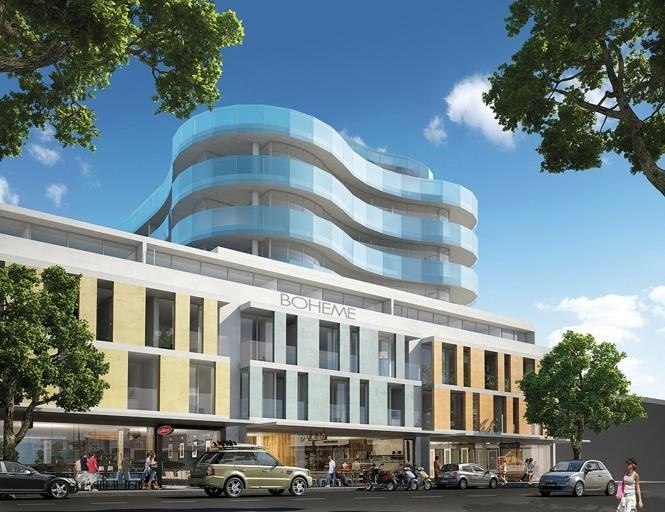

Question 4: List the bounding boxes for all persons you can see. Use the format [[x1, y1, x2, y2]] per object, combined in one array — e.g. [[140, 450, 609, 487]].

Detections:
[[141, 450, 161, 490], [433, 455, 441, 483], [75, 451, 99, 493], [527, 458, 535, 484], [615, 457, 644, 512], [101, 455, 132, 489], [146, 451, 160, 489], [519, 458, 529, 482], [351, 458, 361, 484], [325, 455, 341, 487]]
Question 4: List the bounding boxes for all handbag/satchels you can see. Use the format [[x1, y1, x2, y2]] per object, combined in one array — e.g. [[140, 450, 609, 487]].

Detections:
[[616, 482, 623, 502]]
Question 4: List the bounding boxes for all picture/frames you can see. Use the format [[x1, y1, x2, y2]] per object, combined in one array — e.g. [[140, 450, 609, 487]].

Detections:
[[167, 438, 212, 459]]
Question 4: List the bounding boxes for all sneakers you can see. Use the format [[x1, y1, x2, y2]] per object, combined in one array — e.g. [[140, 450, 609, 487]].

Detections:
[[325, 485, 330, 487]]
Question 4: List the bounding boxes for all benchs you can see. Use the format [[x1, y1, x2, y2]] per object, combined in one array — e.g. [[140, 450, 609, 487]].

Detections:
[[96, 477, 141, 491]]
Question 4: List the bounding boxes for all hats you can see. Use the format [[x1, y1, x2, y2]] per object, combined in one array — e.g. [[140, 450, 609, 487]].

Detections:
[[625, 456, 641, 464]]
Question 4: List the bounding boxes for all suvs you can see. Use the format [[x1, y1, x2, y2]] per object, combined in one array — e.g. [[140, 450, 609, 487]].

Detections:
[[21, 462, 80, 494], [188, 439, 316, 500]]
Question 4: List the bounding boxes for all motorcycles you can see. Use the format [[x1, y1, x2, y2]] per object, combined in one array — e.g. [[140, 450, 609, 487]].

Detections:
[[362, 463, 434, 492]]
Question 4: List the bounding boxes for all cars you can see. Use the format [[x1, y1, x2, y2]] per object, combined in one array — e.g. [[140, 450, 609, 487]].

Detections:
[[0, 459, 70, 499], [536, 459, 616, 496], [435, 462, 500, 490]]
